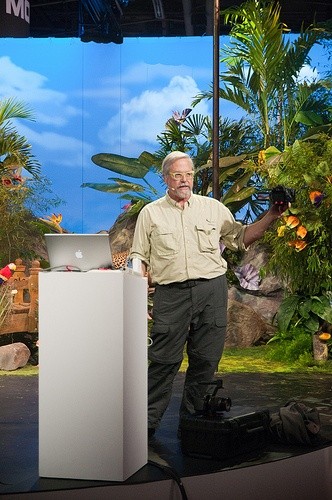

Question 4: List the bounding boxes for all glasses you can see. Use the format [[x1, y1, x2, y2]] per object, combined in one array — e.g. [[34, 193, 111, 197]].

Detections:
[[166, 170, 195, 181]]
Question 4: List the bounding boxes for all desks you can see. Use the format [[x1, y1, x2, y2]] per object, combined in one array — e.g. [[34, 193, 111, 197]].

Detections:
[[38, 270, 150, 482]]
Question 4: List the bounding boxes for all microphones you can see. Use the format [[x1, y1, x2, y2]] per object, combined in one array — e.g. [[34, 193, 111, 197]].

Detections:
[[168, 185, 175, 191]]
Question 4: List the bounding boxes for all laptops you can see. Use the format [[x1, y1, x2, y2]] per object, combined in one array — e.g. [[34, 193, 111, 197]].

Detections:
[[44, 233, 113, 272]]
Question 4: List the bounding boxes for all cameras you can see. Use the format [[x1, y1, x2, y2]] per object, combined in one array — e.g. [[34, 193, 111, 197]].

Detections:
[[269, 185, 296, 205], [194, 379, 232, 416]]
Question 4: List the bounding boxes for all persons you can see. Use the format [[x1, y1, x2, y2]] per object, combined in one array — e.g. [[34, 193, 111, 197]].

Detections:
[[128, 150, 290, 438]]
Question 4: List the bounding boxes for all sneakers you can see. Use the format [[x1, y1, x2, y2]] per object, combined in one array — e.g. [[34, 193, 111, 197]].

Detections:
[[177, 404, 228, 425]]
